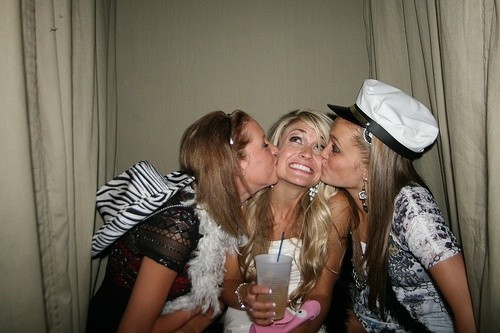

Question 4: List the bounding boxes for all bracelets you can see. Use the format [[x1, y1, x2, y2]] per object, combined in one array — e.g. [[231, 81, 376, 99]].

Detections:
[[233, 282, 249, 310]]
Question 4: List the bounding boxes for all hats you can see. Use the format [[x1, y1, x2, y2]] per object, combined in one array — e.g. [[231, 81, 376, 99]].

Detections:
[[327, 79, 440, 162], [91, 160, 195, 258]]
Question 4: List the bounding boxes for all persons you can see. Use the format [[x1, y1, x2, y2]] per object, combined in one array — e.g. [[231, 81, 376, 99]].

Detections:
[[319, 76, 477, 333], [86, 109, 279, 332], [217, 106, 352, 332]]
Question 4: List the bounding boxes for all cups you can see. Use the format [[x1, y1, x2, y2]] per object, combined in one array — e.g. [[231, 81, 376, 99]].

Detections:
[[253, 253, 293, 320]]
